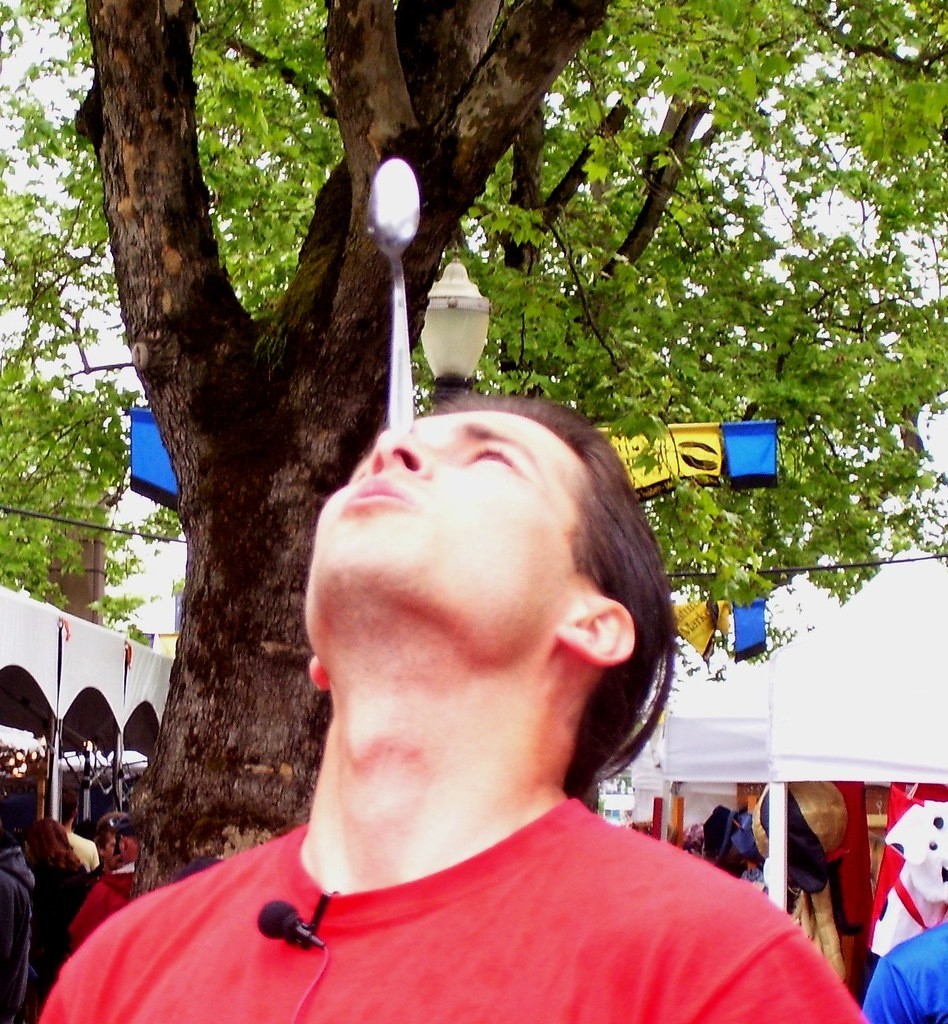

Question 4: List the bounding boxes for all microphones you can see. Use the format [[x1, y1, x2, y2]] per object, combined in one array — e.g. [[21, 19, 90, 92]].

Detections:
[[256, 899, 327, 949]]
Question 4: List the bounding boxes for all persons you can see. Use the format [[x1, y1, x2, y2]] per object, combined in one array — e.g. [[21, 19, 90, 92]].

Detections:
[[36, 395, 948, 1024], [0, 774, 143, 1024]]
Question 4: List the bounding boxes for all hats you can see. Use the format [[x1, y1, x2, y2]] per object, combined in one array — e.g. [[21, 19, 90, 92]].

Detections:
[[703, 805, 735, 857]]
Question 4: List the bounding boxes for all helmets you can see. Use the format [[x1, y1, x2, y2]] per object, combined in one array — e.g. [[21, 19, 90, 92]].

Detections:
[[751, 780, 847, 870]]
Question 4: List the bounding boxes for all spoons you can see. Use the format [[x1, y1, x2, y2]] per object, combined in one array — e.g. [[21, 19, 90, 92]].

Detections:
[[368, 158, 420, 430]]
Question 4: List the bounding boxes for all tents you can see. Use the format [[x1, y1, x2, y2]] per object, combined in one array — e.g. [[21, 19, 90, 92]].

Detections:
[[595, 676, 948, 914]]
